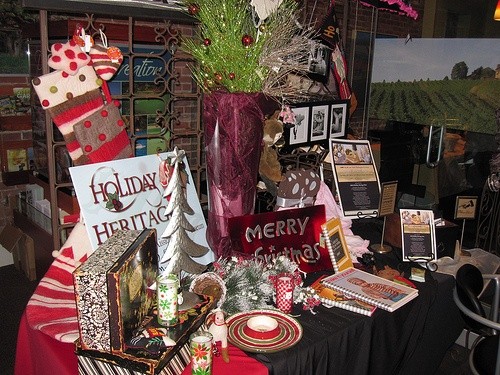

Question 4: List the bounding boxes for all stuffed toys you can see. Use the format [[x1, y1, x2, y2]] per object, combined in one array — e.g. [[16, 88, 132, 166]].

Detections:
[[205, 309, 229, 363], [259, 119, 286, 198]]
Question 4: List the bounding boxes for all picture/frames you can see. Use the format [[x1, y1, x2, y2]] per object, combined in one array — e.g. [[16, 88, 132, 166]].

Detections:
[[287, 103, 310, 148], [311, 102, 330, 145], [332, 100, 351, 140], [288, 27, 331, 83]]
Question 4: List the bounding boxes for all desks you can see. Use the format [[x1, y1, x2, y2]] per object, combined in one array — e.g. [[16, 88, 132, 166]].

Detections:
[[13, 221, 470, 375]]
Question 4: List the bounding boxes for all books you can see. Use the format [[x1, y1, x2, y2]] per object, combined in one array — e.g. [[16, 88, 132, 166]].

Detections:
[[309, 266, 418, 317], [321, 216, 354, 273]]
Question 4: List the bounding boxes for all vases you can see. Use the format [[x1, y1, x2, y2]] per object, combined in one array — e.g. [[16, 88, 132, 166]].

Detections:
[[200, 91, 266, 255]]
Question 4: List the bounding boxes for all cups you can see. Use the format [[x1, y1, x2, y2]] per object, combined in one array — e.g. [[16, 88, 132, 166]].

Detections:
[[156, 273, 179, 327], [276, 273, 294, 314], [189, 331, 213, 375]]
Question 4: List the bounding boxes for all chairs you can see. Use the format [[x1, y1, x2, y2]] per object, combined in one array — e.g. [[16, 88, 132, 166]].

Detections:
[[452, 263, 500, 375]]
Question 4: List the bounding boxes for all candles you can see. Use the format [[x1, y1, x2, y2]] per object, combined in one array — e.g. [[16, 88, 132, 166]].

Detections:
[[190, 332, 213, 375], [156, 273, 180, 327], [275, 273, 295, 313]]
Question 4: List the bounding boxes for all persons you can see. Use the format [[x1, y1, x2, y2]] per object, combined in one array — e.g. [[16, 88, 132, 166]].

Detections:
[[347, 277, 408, 302]]
